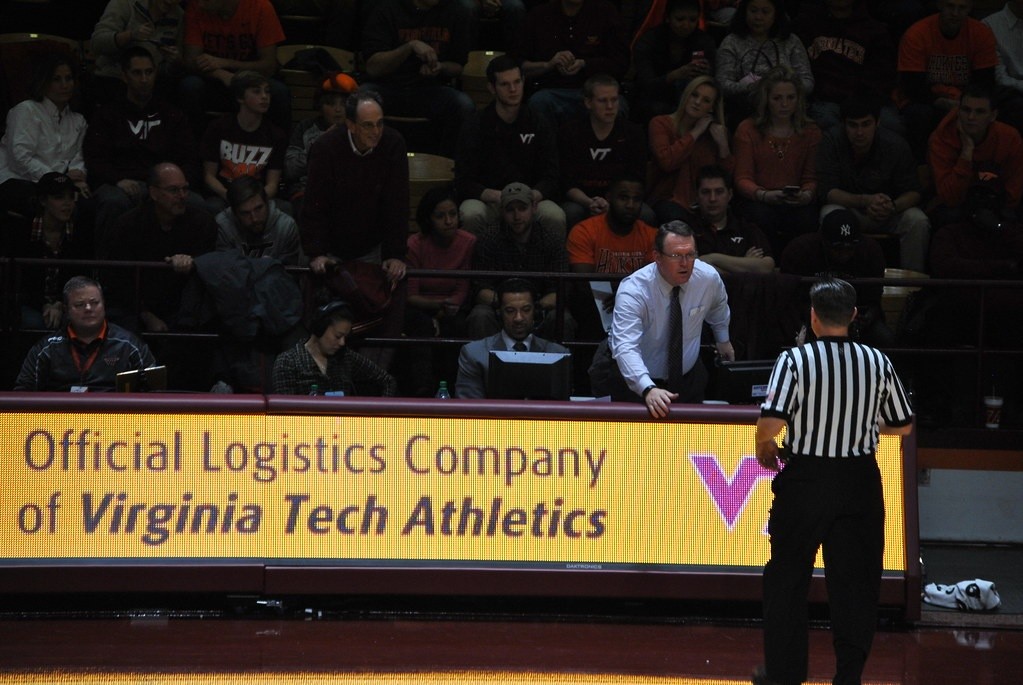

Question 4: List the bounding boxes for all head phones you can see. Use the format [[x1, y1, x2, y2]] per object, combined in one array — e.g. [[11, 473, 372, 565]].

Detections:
[[310, 300, 351, 337], [491, 278, 546, 329]]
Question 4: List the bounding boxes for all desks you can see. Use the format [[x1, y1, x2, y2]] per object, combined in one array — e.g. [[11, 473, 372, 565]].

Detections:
[[0, 390, 920, 628]]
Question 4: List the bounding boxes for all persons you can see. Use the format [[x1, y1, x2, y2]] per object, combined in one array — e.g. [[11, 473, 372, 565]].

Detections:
[[455, 278, 570, 399], [588, 220, 734, 418], [750, 279, 915, 685], [272, 301, 397, 397], [14, 276, 156, 393], [0, 0, 1023, 396]]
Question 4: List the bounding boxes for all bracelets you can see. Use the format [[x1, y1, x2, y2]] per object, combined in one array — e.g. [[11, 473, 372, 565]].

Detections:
[[891, 200, 899, 215], [762, 191, 767, 204]]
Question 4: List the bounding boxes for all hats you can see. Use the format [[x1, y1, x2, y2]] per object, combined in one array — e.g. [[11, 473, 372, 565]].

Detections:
[[500, 181, 533, 209], [35, 172, 81, 196]]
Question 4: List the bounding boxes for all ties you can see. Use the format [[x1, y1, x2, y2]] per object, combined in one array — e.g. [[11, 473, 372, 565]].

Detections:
[[514, 342, 525, 350], [670, 285, 683, 399]]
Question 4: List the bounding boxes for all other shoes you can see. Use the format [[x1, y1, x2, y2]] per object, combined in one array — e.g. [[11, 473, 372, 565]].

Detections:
[[753, 664, 774, 685]]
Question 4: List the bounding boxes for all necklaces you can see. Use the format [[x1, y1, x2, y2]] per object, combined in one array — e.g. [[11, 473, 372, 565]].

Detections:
[[768, 124, 793, 160]]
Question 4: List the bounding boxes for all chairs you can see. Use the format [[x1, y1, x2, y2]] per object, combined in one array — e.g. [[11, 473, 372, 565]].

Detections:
[[878, 267, 930, 338], [0, 33, 508, 236]]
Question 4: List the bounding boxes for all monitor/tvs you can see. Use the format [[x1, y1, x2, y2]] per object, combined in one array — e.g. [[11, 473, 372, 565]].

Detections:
[[719, 358, 777, 406], [489, 350, 571, 401]]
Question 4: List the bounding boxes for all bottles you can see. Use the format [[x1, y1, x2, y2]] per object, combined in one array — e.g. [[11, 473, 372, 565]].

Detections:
[[308, 385, 320, 396], [434, 381, 450, 400]]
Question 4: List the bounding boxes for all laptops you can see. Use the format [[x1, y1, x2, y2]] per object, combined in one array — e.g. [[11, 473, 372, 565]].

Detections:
[[116, 365, 167, 393]]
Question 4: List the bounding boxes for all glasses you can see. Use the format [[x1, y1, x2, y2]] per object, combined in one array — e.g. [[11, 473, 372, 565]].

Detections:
[[151, 182, 188, 193], [661, 248, 698, 263]]
